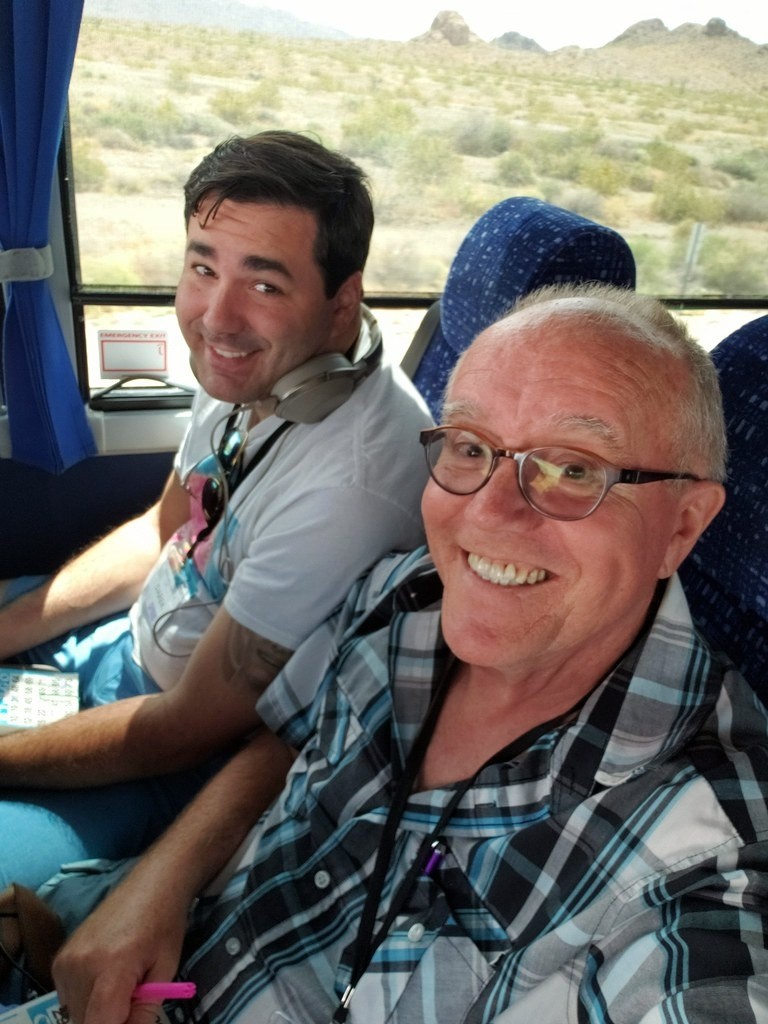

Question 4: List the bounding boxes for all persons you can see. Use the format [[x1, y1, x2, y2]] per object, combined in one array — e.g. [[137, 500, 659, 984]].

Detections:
[[0, 129, 448, 913], [0, 278, 766, 1022]]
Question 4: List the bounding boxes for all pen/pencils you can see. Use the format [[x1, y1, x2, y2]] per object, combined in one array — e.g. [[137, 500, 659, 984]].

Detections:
[[133, 979, 199, 1007]]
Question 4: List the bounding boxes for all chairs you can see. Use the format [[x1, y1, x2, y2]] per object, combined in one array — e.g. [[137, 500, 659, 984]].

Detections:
[[405, 192, 768, 703]]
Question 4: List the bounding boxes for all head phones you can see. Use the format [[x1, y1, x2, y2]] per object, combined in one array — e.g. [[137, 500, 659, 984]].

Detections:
[[270, 301, 384, 422]]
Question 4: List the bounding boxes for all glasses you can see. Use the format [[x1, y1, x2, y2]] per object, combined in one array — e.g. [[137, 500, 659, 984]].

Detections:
[[417, 424, 699, 522], [199, 427, 246, 524]]
